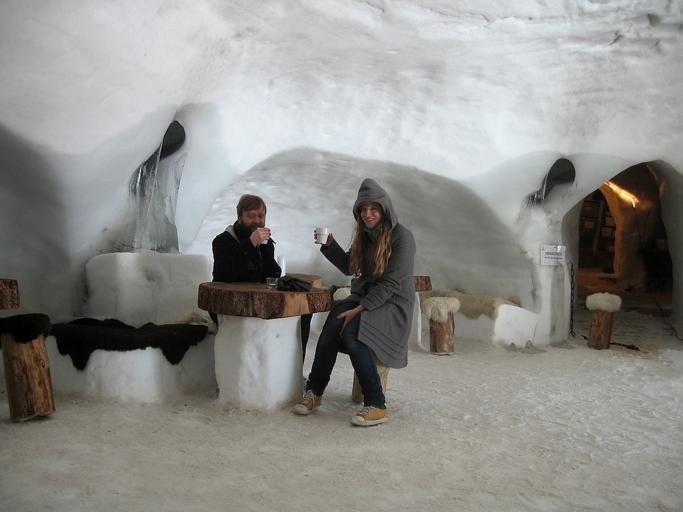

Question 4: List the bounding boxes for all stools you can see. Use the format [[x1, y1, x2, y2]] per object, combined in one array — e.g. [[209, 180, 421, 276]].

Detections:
[[420, 297, 460, 355], [585, 291, 621, 350], [1, 313, 57, 423]]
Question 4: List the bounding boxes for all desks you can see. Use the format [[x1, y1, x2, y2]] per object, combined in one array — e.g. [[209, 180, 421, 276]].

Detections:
[[407, 275, 432, 354], [197, 282, 333, 410]]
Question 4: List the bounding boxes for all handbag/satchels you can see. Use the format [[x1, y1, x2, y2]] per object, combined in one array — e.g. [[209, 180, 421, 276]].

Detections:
[[277, 275, 312, 292]]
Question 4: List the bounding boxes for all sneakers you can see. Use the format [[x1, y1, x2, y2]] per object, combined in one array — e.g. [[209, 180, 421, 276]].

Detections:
[[292, 390, 321, 415], [350, 405, 388, 426]]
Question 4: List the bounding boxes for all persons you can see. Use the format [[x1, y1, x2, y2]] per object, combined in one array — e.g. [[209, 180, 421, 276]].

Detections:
[[208, 194, 313, 390], [295, 178, 416, 426]]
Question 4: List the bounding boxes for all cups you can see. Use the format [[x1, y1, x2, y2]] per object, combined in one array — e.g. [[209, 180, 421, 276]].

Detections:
[[266, 278, 278, 291], [316, 228, 329, 245], [257, 228, 268, 245]]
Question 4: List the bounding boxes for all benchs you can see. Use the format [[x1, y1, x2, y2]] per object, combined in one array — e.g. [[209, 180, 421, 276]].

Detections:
[[420, 290, 538, 346], [44, 321, 217, 402]]
